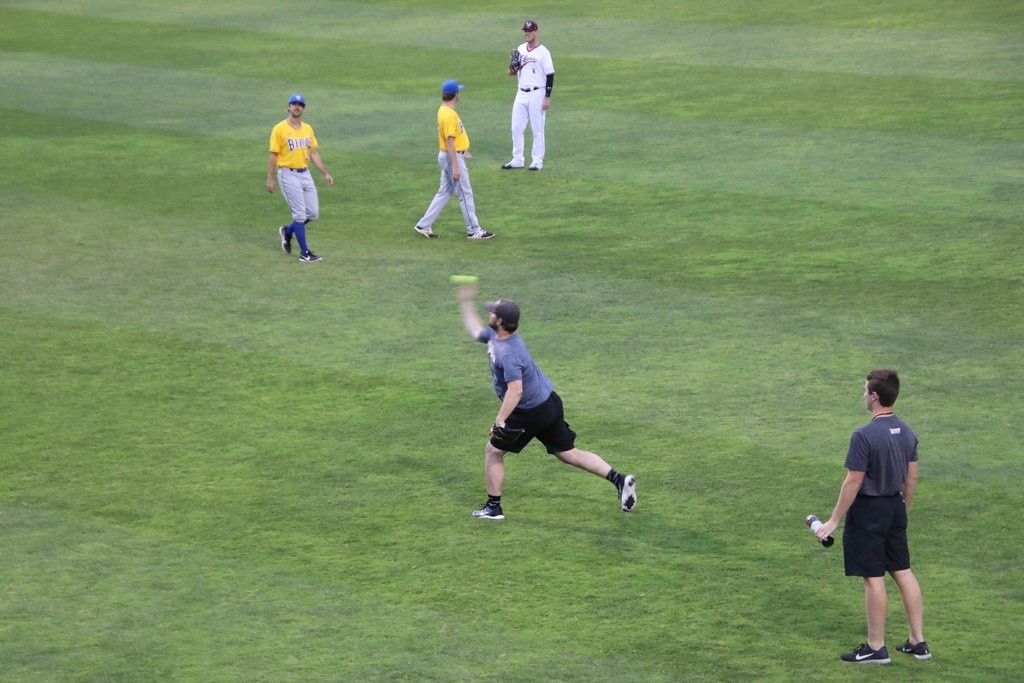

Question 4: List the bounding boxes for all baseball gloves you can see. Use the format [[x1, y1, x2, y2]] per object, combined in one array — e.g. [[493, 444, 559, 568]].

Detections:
[[508, 49, 521, 71], [490, 423, 525, 448]]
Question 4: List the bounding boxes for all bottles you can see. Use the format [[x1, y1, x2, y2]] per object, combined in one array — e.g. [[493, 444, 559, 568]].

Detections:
[[806, 514, 834, 548]]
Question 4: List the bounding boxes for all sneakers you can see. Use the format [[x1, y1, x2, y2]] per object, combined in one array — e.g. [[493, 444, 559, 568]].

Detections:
[[472, 506, 506, 519], [279, 225, 292, 254], [501, 158, 525, 169], [299, 249, 323, 263], [618, 473, 637, 512], [840, 642, 891, 663], [894, 637, 933, 660], [467, 229, 495, 239], [528, 161, 543, 170], [414, 226, 440, 238]]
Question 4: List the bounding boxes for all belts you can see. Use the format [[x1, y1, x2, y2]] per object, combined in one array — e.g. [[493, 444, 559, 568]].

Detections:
[[290, 167, 307, 173], [520, 86, 539, 92], [456, 150, 465, 154]]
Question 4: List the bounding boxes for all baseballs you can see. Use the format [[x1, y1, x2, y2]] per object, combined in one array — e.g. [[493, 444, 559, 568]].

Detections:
[[448, 274, 481, 287]]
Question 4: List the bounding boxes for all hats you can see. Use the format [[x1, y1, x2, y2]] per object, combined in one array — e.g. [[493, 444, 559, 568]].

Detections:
[[288, 94, 306, 105], [521, 20, 538, 31], [442, 80, 464, 93], [484, 299, 520, 323]]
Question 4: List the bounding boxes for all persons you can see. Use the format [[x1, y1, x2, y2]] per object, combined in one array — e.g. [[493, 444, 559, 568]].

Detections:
[[414, 80, 495, 238], [501, 20, 555, 171], [266, 94, 335, 264], [815, 369, 932, 665], [453, 282, 638, 520]]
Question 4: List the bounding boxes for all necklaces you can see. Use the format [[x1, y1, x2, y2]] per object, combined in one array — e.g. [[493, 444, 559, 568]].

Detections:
[[872, 411, 894, 419]]
